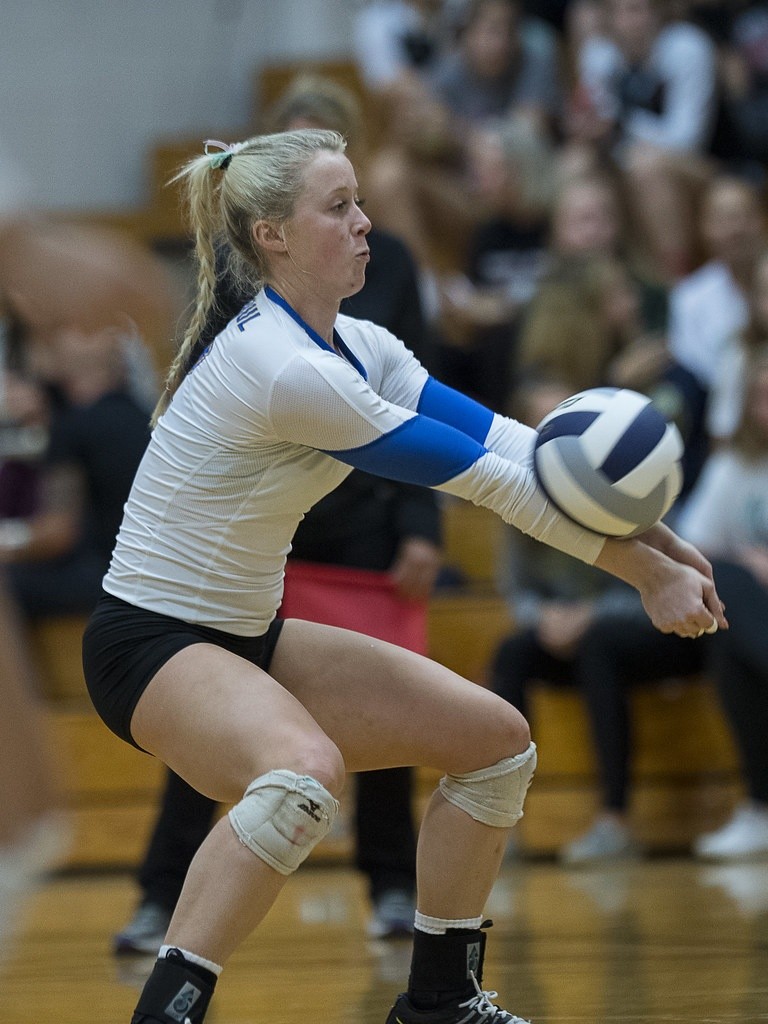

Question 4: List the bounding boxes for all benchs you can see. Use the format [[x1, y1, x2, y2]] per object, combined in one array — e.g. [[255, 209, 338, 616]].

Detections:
[[37, 67, 739, 871]]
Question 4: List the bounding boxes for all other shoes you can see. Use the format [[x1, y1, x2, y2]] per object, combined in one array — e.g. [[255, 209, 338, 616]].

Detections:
[[688, 802, 768, 861], [563, 817, 627, 868], [373, 890, 414, 939], [114, 902, 168, 955]]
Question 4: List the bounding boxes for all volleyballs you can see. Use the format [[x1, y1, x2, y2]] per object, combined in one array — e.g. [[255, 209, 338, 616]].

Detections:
[[531, 387, 684, 539]]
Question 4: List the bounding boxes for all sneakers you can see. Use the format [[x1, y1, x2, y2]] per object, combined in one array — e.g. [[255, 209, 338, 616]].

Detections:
[[385, 970, 527, 1024]]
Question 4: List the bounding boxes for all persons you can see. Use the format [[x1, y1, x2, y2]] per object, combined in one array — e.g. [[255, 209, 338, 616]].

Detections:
[[83, 130, 729, 1024], [0, 0, 768, 961]]
